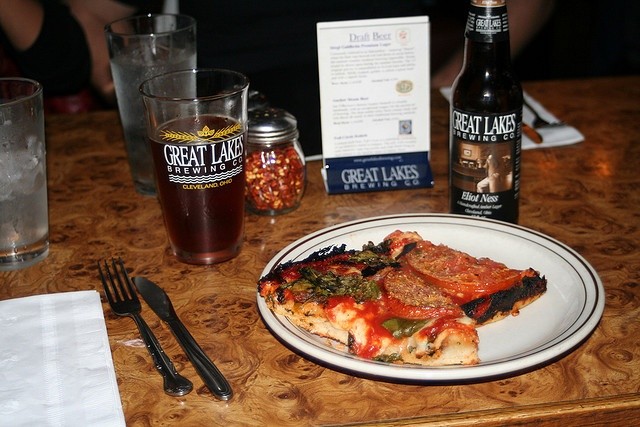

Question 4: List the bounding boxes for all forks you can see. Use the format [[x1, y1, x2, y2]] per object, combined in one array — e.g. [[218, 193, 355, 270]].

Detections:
[[523, 86, 567, 130], [97, 259, 192, 396]]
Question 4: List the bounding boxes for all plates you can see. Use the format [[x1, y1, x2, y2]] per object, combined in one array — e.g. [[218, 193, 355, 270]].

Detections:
[[259, 212, 607, 382]]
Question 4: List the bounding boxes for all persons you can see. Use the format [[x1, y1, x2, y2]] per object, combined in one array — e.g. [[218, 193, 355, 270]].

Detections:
[[68, 0, 556, 110], [1, 0, 91, 95]]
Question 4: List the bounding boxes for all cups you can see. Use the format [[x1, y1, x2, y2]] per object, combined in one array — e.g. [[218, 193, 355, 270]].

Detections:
[[0, 76, 48, 271], [106, 16, 199, 195], [141, 69, 251, 264]]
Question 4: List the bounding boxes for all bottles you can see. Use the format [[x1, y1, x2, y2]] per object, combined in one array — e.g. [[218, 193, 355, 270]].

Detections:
[[448, 0, 522, 223], [244, 108, 306, 217]]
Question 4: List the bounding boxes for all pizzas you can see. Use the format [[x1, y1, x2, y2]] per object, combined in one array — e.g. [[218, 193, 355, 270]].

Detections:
[[372, 230, 547, 326], [256, 245, 479, 367]]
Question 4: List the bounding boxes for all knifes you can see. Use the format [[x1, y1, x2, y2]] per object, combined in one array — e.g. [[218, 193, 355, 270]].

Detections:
[[523, 123, 544, 144], [133, 277, 233, 400]]
